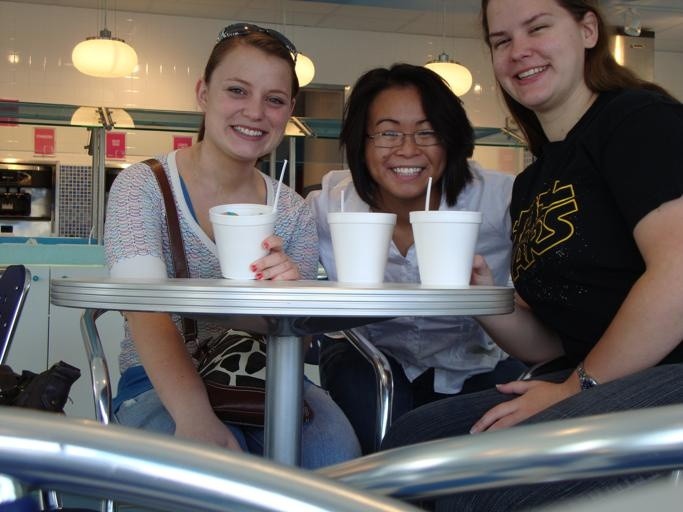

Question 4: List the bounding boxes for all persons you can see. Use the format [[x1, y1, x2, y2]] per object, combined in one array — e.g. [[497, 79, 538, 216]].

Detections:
[[99, 22, 360, 474], [306, 60, 528, 460], [373, 0, 683, 454]]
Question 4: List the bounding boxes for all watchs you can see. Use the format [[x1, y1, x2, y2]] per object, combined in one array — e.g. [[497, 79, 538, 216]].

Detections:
[[574, 361, 598, 391]]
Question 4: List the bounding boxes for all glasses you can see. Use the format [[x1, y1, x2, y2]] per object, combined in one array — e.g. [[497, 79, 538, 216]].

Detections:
[[368, 130, 439, 148], [215, 22, 299, 66]]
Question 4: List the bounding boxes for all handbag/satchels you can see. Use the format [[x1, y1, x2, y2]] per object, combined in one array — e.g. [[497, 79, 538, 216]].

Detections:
[[198, 381, 312, 428]]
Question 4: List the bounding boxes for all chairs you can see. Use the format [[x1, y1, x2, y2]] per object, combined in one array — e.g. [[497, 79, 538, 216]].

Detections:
[[0, 264, 31, 366], [314, 403, 682, 512], [0, 406, 425, 512]]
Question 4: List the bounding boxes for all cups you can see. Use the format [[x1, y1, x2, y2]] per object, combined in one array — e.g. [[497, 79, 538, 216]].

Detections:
[[325, 211, 399, 288], [208, 203, 279, 282], [407, 210, 483, 286]]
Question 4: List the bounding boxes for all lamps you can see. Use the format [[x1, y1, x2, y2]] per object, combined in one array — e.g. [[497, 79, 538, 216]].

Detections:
[[71, 107, 135, 127], [71, 0, 138, 78], [289, 0, 315, 88], [423, 0, 472, 97]]
